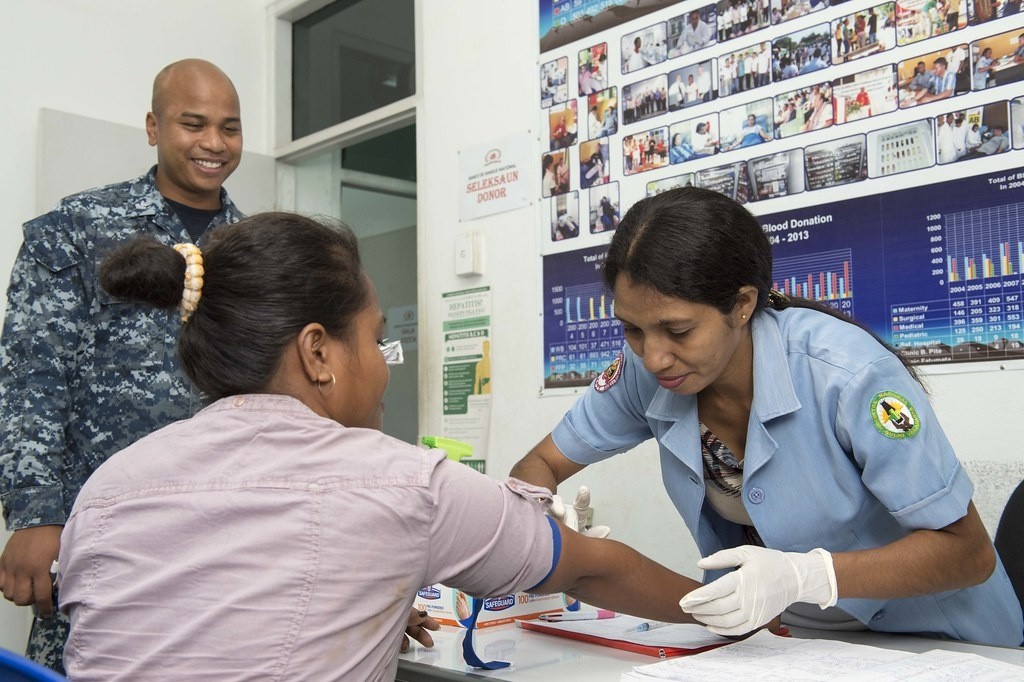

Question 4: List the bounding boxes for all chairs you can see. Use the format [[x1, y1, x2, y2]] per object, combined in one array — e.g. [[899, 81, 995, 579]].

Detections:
[[994, 478, 1023, 648]]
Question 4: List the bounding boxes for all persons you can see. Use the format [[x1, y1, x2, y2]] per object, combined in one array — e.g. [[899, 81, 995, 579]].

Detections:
[[0, 59, 249, 675], [55, 213, 782, 681], [594, 195, 620, 232], [556, 210, 578, 242], [541, 0, 1024, 198], [507, 188, 1024, 649]]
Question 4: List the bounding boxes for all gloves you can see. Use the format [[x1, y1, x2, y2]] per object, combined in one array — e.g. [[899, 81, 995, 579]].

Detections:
[[680, 546, 838, 636], [542, 487, 610, 538]]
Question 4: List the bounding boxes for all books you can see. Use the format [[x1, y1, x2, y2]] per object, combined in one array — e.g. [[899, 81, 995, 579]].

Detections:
[[621, 629, 1024, 682]]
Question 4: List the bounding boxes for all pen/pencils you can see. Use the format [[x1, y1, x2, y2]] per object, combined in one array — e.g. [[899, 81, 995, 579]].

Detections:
[[537, 609, 623, 623]]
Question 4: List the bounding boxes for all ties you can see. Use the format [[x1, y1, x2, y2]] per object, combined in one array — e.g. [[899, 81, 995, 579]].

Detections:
[[679, 86, 683, 100]]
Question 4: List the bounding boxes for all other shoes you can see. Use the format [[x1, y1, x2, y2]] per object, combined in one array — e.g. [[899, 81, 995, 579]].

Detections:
[[951, 26, 956, 32]]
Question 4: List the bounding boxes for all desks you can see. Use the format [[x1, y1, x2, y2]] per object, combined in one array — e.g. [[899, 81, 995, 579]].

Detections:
[[394, 602, 1023, 682]]
